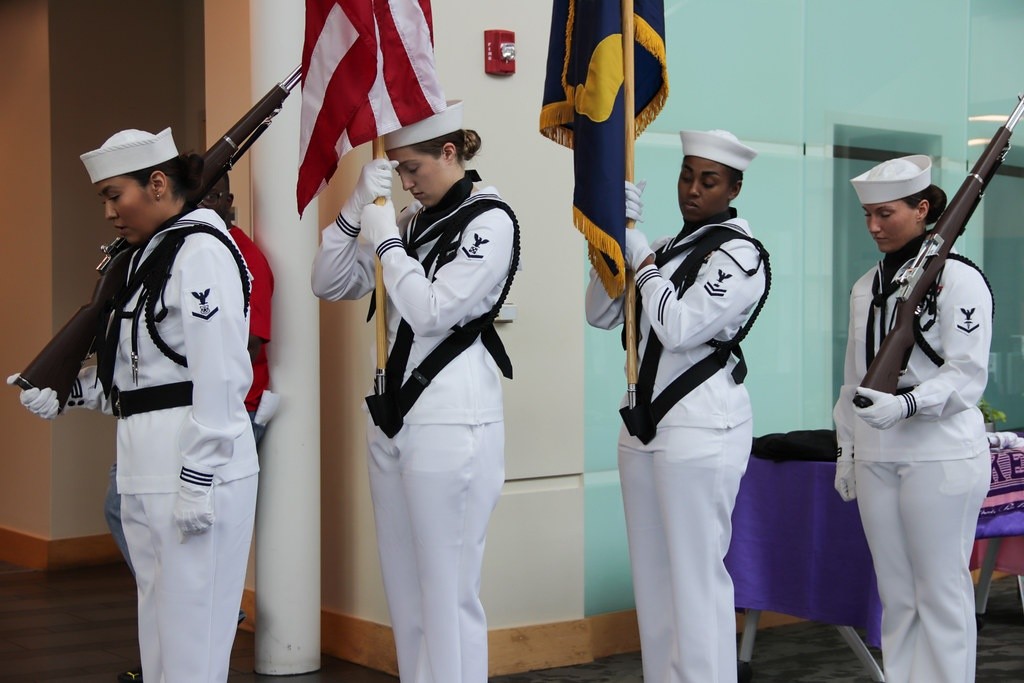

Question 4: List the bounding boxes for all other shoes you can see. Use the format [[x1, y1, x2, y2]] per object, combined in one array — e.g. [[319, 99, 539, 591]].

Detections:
[[238, 610, 246, 626], [118, 668, 143, 683]]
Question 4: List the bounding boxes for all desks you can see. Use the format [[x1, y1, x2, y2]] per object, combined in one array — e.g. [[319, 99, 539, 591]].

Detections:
[[727, 431, 1024, 683]]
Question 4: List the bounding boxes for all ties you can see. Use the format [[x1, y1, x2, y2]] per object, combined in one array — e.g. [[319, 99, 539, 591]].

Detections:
[[865, 269, 900, 372], [97, 234, 168, 401], [621, 237, 699, 352], [366, 207, 453, 323]]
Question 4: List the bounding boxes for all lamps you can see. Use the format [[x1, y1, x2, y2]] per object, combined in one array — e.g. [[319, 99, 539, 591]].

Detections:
[[484, 29, 517, 76]]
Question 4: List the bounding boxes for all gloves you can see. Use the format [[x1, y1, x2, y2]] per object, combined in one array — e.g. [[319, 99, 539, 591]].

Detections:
[[835, 462, 857, 502], [853, 387, 903, 430], [361, 200, 399, 249], [625, 179, 647, 223], [7, 372, 65, 421], [173, 490, 214, 544], [626, 227, 655, 273], [342, 158, 399, 223]]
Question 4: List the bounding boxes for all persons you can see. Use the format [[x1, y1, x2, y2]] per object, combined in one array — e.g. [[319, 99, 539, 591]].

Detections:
[[6, 126, 273, 683], [832, 154, 995, 683], [310, 100, 521, 683], [584, 128, 771, 683]]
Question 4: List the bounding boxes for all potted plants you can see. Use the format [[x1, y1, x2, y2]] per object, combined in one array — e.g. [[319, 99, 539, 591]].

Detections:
[[978, 395, 1008, 433]]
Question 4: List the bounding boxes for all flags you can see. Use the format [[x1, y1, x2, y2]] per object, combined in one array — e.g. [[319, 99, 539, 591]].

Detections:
[[296, 0, 449, 220], [539, 0, 668, 300]]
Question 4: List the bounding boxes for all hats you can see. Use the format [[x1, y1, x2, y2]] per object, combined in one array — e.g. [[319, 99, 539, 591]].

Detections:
[[849, 154, 932, 205], [680, 129, 758, 171], [384, 99, 464, 152], [79, 126, 180, 184]]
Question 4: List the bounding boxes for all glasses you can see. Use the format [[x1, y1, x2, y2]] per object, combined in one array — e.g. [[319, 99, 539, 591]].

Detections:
[[200, 191, 229, 209]]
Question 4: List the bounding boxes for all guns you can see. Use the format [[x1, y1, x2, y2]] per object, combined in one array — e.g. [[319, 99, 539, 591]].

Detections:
[[13, 64, 303, 414], [850, 92, 1024, 410]]
